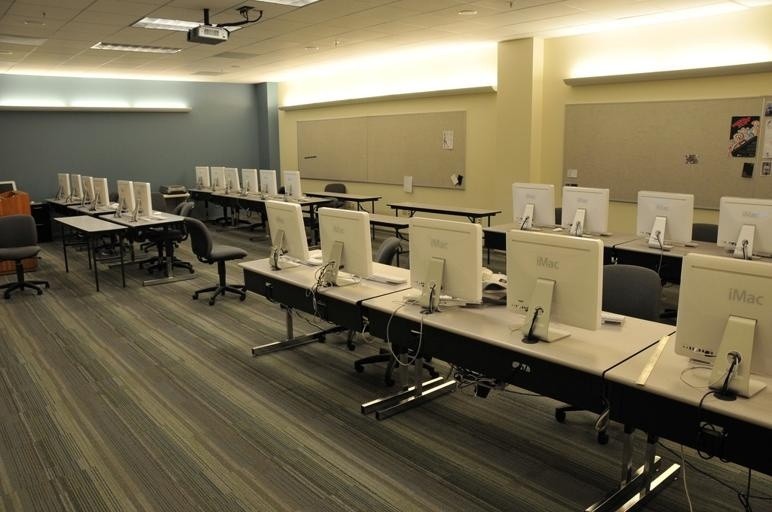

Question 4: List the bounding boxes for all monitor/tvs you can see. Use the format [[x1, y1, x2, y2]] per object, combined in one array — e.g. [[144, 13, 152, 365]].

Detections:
[[194, 166, 312, 201], [674, 253, 771, 401], [409, 218, 484, 310], [636, 190, 694, 251], [512, 182, 555, 230], [562, 186, 610, 237], [264, 200, 309, 270], [505, 229, 603, 343], [317, 208, 374, 286], [716, 197, 772, 259], [56, 171, 153, 222]]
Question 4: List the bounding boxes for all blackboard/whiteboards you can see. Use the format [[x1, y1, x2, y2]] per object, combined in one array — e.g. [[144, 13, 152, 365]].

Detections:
[[562, 95, 772, 211], [296, 110, 467, 190]]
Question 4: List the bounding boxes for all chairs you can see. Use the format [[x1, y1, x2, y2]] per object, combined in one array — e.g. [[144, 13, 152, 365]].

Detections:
[[0, 214, 50, 297]]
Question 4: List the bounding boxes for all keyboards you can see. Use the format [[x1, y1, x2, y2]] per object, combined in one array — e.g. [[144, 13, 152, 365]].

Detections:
[[152, 214, 167, 220], [309, 251, 323, 265], [370, 261, 407, 285], [602, 310, 626, 325], [109, 202, 119, 209], [685, 241, 699, 248]]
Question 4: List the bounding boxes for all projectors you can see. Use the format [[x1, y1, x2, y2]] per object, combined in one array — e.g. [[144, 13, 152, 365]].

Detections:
[[187, 26, 229, 45]]
